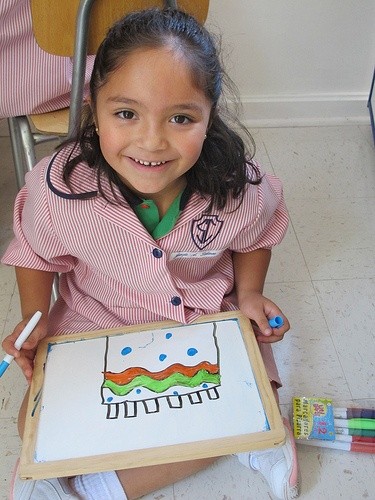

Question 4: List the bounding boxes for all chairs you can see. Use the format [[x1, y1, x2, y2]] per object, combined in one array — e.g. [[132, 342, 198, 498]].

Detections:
[[7, 0, 211, 302]]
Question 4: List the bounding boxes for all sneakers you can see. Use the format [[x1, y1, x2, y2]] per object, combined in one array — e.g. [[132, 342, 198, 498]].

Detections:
[[10, 458, 81, 500], [239, 417, 300, 500]]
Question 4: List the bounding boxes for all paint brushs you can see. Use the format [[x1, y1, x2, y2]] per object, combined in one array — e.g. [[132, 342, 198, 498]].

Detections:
[[293, 402, 375, 453], [0, 311, 42, 376]]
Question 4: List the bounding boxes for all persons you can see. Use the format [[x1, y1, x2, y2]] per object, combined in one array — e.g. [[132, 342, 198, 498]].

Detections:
[[1, 10, 300, 500]]
